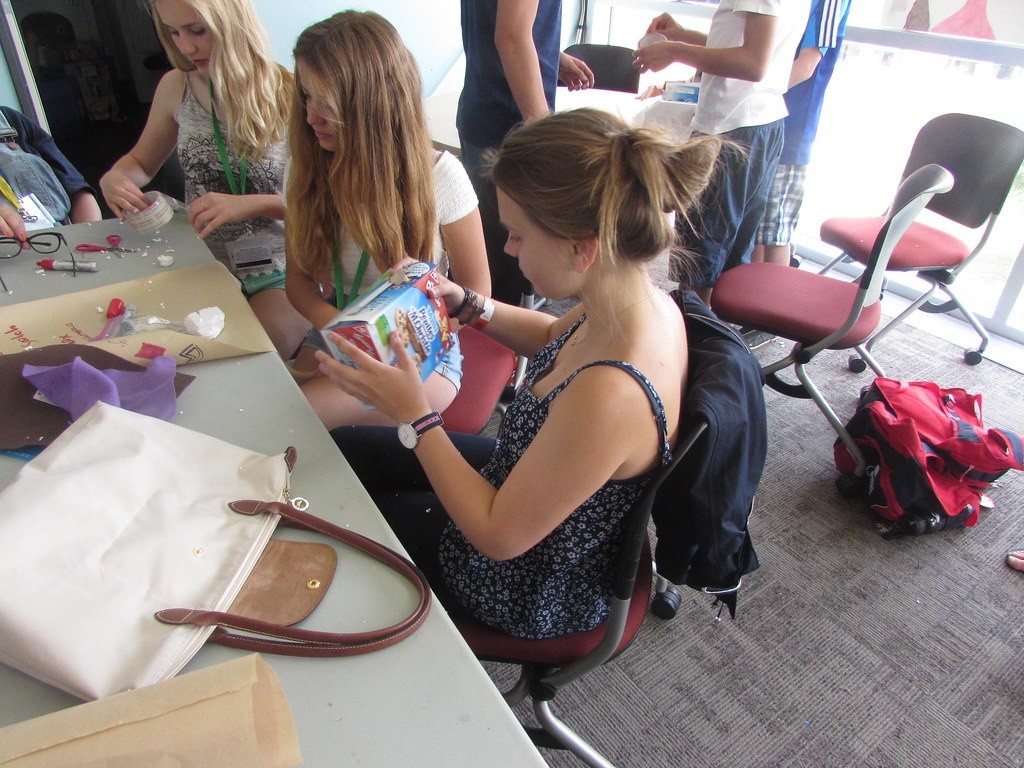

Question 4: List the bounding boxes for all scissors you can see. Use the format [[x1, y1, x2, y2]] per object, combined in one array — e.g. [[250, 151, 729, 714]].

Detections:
[[76, 234, 137, 258]]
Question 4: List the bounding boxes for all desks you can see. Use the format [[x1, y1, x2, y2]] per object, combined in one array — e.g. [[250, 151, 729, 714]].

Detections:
[[555, 87, 698, 145], [0, 212, 551, 768]]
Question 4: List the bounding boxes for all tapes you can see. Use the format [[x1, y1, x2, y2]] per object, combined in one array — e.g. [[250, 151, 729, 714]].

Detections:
[[122, 190, 191, 235], [638, 32, 667, 49]]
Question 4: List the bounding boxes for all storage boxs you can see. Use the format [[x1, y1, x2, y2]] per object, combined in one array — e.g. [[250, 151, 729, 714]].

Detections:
[[318, 260, 456, 412], [661, 79, 700, 103]]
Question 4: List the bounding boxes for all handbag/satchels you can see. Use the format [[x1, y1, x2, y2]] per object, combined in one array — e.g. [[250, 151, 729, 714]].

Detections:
[[0, 399, 433, 701]]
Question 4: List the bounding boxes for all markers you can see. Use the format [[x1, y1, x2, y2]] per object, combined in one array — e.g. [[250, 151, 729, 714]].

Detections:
[[36, 259, 98, 272]]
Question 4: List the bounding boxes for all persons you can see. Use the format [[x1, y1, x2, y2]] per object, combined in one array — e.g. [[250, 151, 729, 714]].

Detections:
[[0, 106, 102, 250], [99, 0, 312, 362], [281, 11, 492, 433], [456, 0, 594, 304], [632, 0, 810, 309], [750, 0, 851, 266], [315, 108, 725, 640]]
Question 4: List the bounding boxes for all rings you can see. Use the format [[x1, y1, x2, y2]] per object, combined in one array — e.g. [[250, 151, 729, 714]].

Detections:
[[201, 202, 206, 210]]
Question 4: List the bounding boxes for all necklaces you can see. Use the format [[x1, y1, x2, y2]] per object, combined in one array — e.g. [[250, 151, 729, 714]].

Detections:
[[570, 289, 654, 346]]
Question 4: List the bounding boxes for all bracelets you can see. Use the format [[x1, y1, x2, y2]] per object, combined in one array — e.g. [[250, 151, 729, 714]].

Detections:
[[448, 287, 495, 330]]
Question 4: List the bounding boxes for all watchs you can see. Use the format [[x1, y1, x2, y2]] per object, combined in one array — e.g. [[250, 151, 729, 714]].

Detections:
[[398, 411, 444, 449]]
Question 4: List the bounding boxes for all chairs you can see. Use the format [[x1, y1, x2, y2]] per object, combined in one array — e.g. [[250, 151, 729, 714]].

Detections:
[[557, 43, 641, 95], [449, 289, 769, 768], [710, 163, 956, 498], [816, 113, 1024, 375], [439, 222, 537, 436]]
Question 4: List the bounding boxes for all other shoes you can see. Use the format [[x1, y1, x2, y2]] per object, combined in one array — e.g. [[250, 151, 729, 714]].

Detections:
[[727, 320, 756, 336]]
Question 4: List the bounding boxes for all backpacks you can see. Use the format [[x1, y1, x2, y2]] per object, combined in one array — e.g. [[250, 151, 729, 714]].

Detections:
[[833, 377, 1024, 541]]
